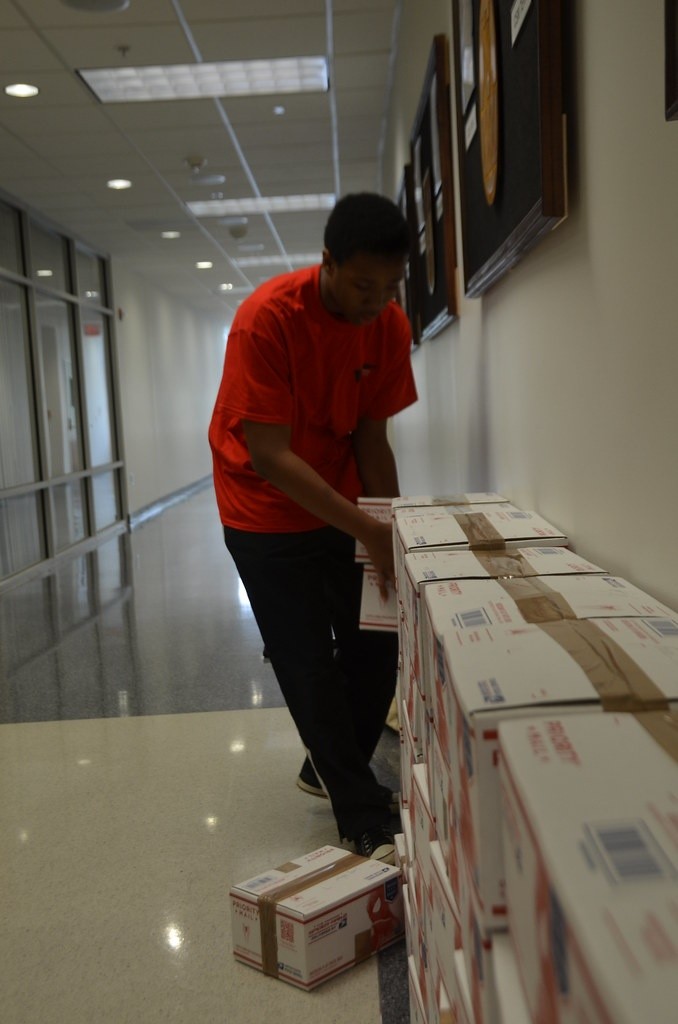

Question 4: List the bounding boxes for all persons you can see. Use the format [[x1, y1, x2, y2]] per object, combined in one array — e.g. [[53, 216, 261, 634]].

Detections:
[[206, 192, 419, 865]]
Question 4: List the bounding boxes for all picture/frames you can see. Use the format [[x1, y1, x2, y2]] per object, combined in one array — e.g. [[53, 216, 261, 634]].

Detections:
[[397, 0, 678, 352]]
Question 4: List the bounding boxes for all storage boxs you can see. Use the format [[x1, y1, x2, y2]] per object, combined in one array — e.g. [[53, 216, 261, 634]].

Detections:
[[230, 488, 678, 1024]]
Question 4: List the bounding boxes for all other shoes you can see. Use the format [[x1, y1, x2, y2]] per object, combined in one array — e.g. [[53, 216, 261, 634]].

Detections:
[[295, 759, 396, 809], [354, 828, 398, 867]]
